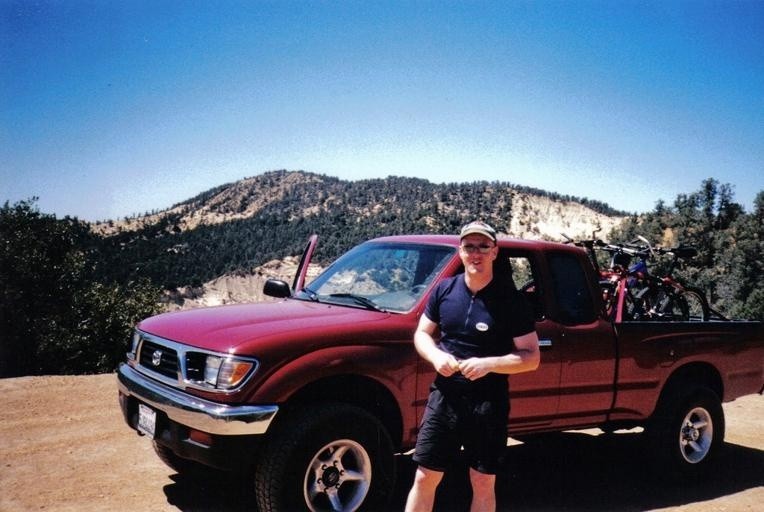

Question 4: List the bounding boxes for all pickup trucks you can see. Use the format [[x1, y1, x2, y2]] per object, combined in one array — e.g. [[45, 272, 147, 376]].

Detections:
[[116, 233, 764, 512]]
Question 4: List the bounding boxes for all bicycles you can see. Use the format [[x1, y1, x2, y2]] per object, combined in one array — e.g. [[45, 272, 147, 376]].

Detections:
[[520, 229, 709, 321]]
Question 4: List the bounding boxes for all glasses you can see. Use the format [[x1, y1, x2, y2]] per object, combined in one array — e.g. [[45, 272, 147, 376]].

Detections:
[[462, 244, 490, 253]]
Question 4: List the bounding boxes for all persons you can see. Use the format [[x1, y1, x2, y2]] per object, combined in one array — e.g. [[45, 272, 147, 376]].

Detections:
[[404, 220, 540, 511]]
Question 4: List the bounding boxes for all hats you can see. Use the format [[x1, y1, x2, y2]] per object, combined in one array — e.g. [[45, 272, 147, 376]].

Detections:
[[460, 221, 496, 243]]
